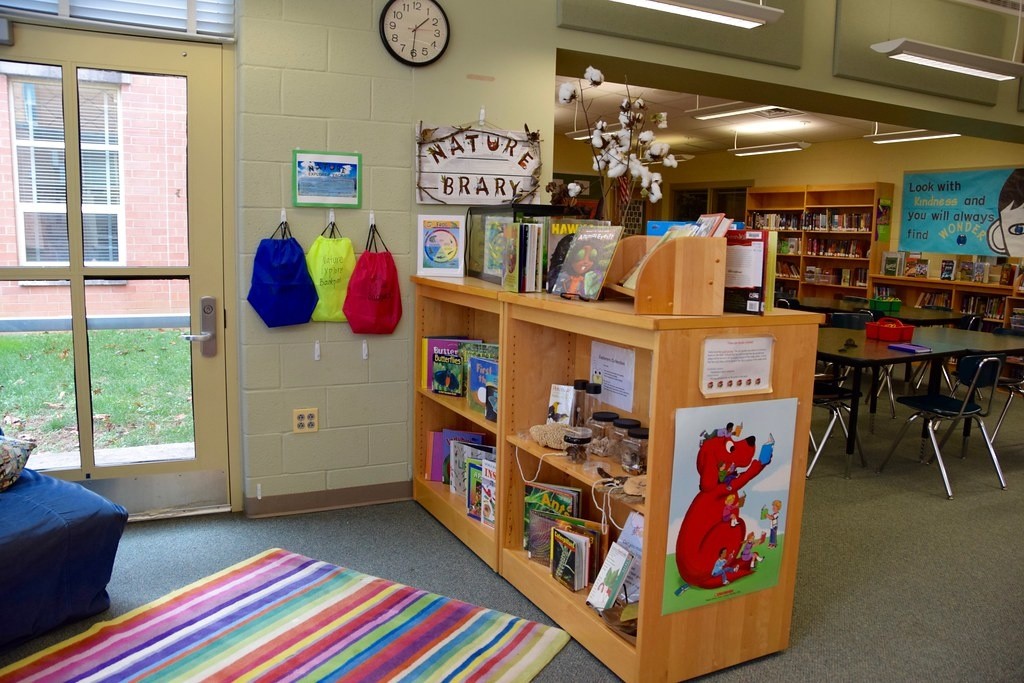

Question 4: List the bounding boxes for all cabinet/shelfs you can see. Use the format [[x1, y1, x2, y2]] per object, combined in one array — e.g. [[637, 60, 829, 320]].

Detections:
[[407, 274, 501, 574], [745, 185, 805, 305], [1002, 297, 1023, 383], [867, 274, 953, 326], [801, 181, 895, 302], [951, 285, 1012, 334], [500, 291, 826, 682]]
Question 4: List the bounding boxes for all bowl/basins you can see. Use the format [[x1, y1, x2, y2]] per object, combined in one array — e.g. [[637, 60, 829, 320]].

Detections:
[[865, 317, 916, 341], [988, 274, 1000, 284], [868, 295, 902, 311]]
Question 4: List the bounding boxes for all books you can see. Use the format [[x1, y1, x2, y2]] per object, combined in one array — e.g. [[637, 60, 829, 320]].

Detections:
[[914, 292, 951, 308], [748, 211, 802, 298], [523, 481, 645, 617], [960, 296, 1006, 319], [880, 250, 929, 278], [874, 287, 896, 297], [417, 215, 768, 316], [802, 211, 873, 284], [546, 385, 577, 426], [421, 336, 499, 423], [426, 429, 497, 528], [940, 260, 1024, 290]]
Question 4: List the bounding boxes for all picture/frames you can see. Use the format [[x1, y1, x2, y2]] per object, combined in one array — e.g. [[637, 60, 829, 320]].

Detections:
[[293, 148, 362, 208], [881, 251, 905, 277]]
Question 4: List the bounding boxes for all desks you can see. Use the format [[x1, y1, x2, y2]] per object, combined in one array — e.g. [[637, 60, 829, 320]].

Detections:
[[788, 296, 968, 327], [816, 328, 1024, 455]]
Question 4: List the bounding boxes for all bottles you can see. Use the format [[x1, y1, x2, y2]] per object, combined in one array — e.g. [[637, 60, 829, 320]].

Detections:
[[1000, 263, 1012, 285], [955, 272, 961, 280], [564, 379, 649, 475]]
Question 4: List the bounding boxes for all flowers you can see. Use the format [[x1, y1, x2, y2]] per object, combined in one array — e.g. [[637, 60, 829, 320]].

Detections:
[[558, 65, 678, 202]]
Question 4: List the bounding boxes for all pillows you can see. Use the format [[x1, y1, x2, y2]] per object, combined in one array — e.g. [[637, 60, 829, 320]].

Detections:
[[0, 436, 39, 490]]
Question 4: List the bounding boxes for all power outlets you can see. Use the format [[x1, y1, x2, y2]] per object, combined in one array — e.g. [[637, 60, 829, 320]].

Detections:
[[293, 408, 319, 432]]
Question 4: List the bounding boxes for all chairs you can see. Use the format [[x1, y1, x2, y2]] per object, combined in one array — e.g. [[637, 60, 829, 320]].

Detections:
[[773, 294, 1024, 499], [0, 430, 128, 645]]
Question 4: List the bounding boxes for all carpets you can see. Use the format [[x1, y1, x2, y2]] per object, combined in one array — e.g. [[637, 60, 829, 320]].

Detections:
[[0, 547, 569, 683]]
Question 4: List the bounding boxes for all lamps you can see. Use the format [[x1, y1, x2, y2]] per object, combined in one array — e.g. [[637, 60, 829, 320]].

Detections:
[[727, 127, 813, 157], [862, 123, 961, 145], [870, 34, 1024, 82], [682, 94, 781, 122], [609, 0, 784, 30]]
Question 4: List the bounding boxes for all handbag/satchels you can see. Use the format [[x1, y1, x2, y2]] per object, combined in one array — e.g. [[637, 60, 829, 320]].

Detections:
[[246, 222, 320, 328], [342, 224, 402, 335], [306, 222, 357, 323]]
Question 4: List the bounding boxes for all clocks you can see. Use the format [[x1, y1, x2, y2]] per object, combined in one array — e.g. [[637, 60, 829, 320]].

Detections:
[[379, 1, 451, 67]]
